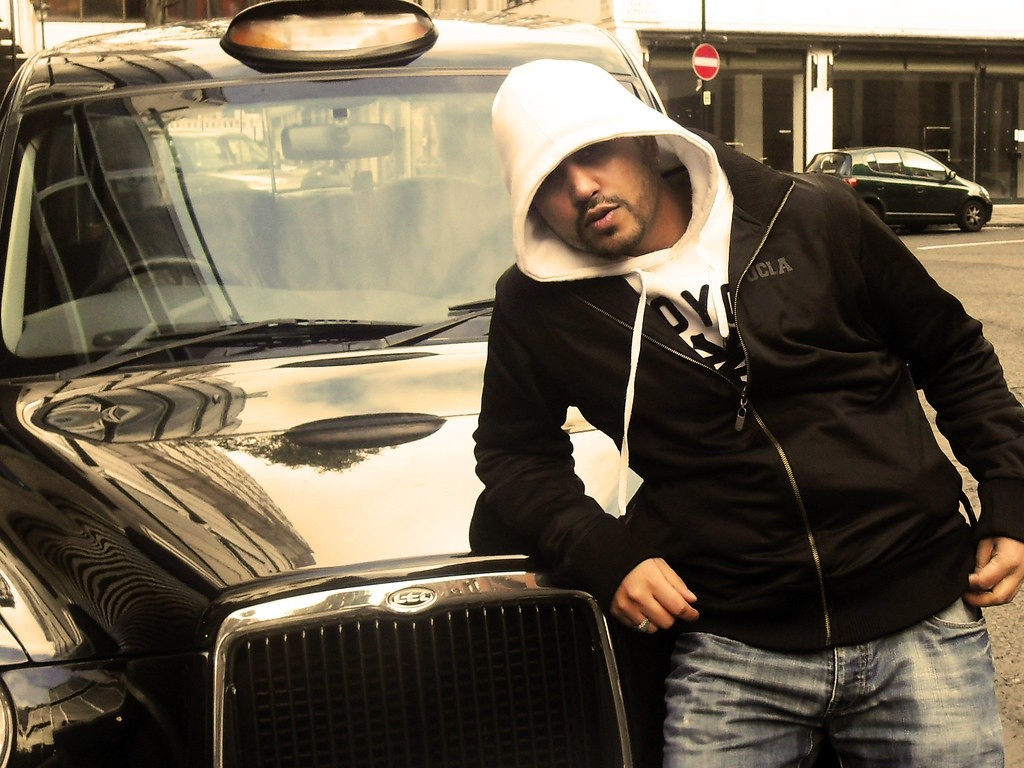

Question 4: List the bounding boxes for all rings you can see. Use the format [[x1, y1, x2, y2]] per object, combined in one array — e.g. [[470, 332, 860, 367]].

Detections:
[[637, 618, 650, 633]]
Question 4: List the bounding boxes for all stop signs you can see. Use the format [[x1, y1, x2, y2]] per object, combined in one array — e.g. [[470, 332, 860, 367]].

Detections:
[[692, 45, 720, 82]]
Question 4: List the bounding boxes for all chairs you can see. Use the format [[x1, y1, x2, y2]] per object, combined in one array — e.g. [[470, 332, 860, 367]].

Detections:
[[87, 172, 513, 304]]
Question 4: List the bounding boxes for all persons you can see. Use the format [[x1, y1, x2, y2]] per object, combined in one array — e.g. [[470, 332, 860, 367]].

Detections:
[[471, 59, 1024, 768]]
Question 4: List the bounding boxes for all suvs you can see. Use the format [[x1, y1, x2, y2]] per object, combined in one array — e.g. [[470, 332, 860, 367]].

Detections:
[[0, 0, 690, 768], [804, 147, 994, 234]]
